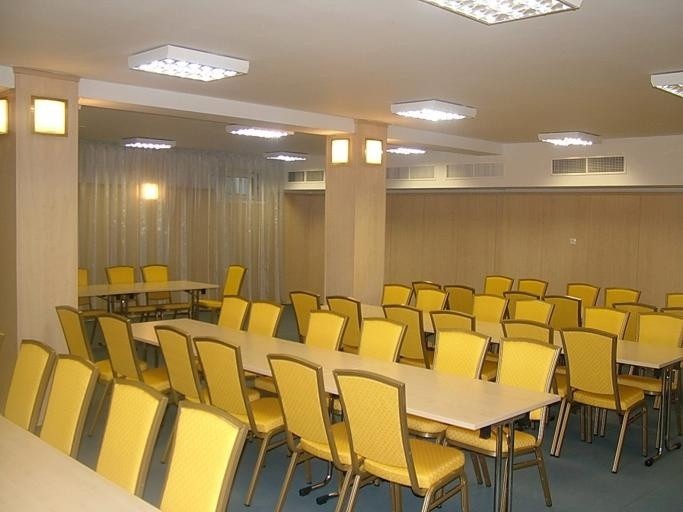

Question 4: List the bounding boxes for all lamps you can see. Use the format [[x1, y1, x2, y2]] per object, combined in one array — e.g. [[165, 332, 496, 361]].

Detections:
[[390, 99, 479, 122], [264, 151, 310, 163], [224, 124, 288, 140], [537, 131, 601, 148], [420, 1, 585, 27], [649, 71, 683, 99], [122, 136, 177, 150], [127, 44, 251, 82]]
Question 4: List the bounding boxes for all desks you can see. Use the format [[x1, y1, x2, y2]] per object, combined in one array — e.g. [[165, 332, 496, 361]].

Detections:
[[77, 280, 221, 315], [0, 417, 164, 512], [319, 298, 683, 467], [126, 316, 564, 509]]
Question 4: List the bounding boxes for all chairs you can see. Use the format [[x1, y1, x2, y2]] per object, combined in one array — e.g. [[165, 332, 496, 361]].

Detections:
[[555, 327, 648, 474], [661, 307, 682, 319], [483, 275, 514, 298], [381, 284, 414, 305], [324, 317, 408, 418], [290, 290, 320, 343], [97, 314, 171, 400], [154, 325, 263, 463], [403, 328, 491, 508], [438, 336, 562, 512], [514, 299, 556, 326], [430, 310, 496, 381], [627, 312, 683, 449], [106, 265, 157, 334], [382, 304, 435, 370], [5, 339, 56, 433], [255, 309, 350, 396], [333, 369, 470, 512], [195, 264, 249, 322], [503, 289, 538, 320], [162, 398, 248, 512], [664, 292, 682, 309], [217, 294, 251, 331], [249, 300, 285, 337], [567, 281, 601, 308], [327, 295, 361, 353], [518, 278, 549, 301], [55, 305, 146, 437], [267, 353, 380, 512], [78, 267, 105, 346], [614, 300, 657, 341], [445, 284, 474, 315], [414, 288, 449, 310], [603, 286, 642, 308], [141, 260, 191, 320], [95, 378, 168, 498], [542, 294, 583, 328], [582, 305, 631, 437], [473, 294, 510, 324], [39, 353, 100, 460], [501, 319, 586, 448], [412, 281, 440, 292], [193, 337, 285, 507]]
